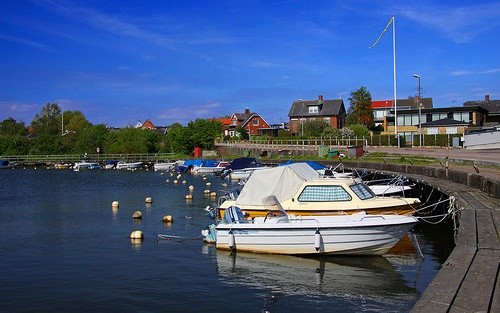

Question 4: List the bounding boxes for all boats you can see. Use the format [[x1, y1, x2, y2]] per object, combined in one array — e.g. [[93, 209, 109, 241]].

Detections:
[[152, 154, 422, 258], [0, 159, 16, 169], [54, 159, 144, 171]]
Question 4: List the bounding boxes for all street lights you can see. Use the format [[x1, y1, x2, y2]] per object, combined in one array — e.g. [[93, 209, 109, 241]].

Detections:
[[412, 73, 422, 149], [298, 98, 304, 138]]
